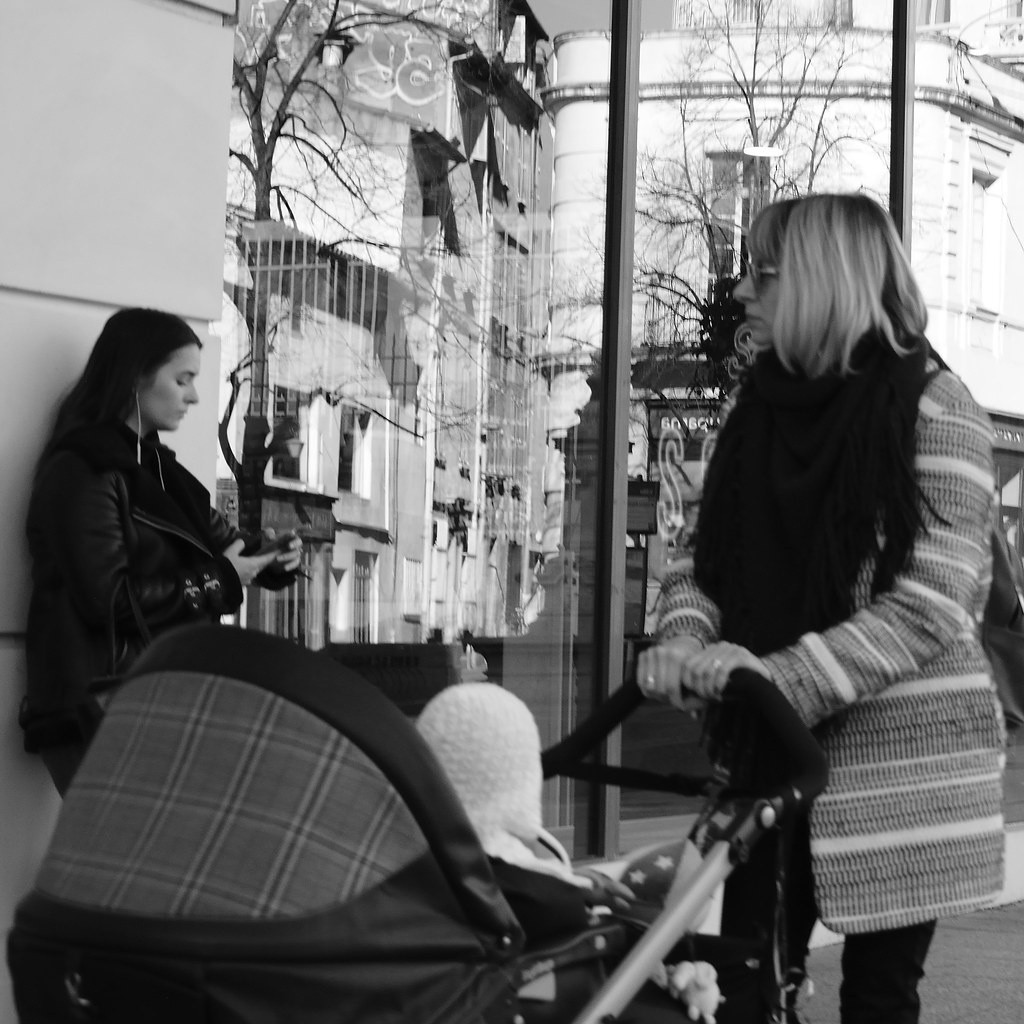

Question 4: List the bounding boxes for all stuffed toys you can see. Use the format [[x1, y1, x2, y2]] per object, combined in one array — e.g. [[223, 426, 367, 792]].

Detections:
[[666, 961, 726, 1024]]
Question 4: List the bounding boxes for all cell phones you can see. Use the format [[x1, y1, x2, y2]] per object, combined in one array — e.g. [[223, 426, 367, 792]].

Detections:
[[255, 529, 296, 556]]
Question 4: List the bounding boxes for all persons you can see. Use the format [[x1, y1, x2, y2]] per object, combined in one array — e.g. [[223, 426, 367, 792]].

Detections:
[[415, 193, 1007, 1024], [17, 308, 303, 801]]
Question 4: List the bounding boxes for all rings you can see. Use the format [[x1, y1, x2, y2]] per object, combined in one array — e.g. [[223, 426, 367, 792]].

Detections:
[[713, 659, 722, 669], [645, 675, 655, 691], [299, 548, 303, 553]]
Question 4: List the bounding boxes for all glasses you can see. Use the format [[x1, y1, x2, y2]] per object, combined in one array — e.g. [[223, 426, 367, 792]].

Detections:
[[744, 259, 778, 292]]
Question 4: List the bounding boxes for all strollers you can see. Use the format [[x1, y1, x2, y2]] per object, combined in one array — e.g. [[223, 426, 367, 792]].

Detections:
[[1, 623, 833, 1024]]
